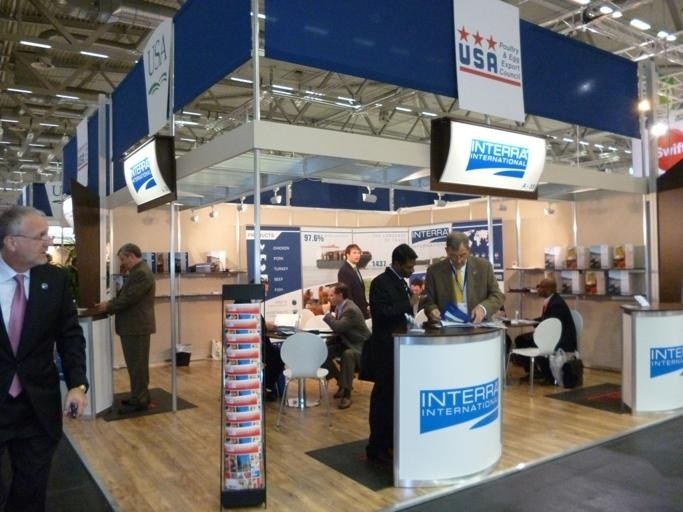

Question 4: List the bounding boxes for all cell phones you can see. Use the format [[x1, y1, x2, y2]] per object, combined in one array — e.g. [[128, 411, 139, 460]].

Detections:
[[70, 401, 76, 418]]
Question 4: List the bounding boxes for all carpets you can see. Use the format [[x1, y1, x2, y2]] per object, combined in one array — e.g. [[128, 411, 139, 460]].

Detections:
[[542, 382, 632, 417], [101, 385, 197, 423], [304, 438, 394, 492]]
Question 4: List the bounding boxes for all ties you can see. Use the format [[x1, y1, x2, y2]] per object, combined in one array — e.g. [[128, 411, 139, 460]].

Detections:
[[7, 275, 28, 398], [454, 269, 463, 305]]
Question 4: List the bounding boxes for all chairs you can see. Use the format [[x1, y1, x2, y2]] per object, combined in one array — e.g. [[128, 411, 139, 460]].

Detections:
[[505, 316, 563, 396], [276, 331, 333, 429], [570, 309, 584, 333]]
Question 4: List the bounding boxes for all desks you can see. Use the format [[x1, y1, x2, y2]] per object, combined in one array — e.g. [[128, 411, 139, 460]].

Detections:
[[503, 317, 540, 389], [264, 326, 339, 410]]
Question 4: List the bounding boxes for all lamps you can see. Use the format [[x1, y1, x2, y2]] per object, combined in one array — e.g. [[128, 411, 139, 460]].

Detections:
[[544, 201, 555, 215], [362, 187, 378, 204], [434, 193, 447, 208], [190, 196, 247, 223], [270, 186, 282, 205]]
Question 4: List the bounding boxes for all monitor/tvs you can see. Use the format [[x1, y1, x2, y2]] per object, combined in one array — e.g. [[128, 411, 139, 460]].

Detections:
[[633, 292, 650, 308]]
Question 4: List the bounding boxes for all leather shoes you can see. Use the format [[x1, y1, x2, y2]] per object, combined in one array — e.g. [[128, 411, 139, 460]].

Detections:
[[333, 385, 352, 409], [117, 399, 149, 414], [520, 371, 554, 386], [365, 445, 393, 464]]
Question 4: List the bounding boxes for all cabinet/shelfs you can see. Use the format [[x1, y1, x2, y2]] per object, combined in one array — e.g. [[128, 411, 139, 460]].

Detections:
[[505, 266, 647, 301]]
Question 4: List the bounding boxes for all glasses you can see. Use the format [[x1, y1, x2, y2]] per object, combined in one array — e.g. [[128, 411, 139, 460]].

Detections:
[[10, 234, 55, 242]]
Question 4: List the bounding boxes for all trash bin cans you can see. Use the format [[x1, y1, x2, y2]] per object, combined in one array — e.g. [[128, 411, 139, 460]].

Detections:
[[176, 344, 192, 366]]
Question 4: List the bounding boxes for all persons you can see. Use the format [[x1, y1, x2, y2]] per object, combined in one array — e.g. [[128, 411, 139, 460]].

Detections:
[[408, 277, 424, 297], [422, 231, 506, 325], [338, 243, 369, 320], [1, 205, 90, 512], [320, 282, 371, 410], [358, 243, 429, 471], [233, 275, 286, 402], [514, 278, 577, 386], [87, 244, 156, 415], [46, 253, 52, 266], [71, 257, 80, 308], [495, 306, 512, 365]]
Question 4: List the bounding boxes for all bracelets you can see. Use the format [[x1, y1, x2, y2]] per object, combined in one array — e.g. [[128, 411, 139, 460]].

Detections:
[[74, 384, 87, 393]]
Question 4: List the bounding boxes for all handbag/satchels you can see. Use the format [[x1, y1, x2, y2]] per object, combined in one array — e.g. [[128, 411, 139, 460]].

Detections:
[[562, 357, 583, 387], [548, 348, 568, 387]]
